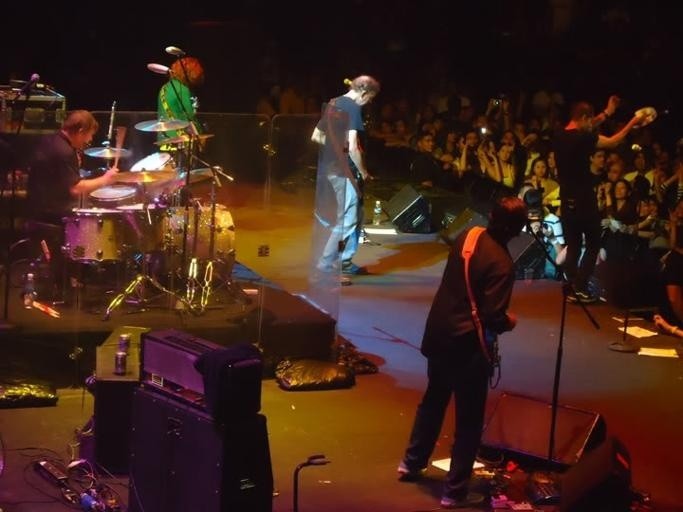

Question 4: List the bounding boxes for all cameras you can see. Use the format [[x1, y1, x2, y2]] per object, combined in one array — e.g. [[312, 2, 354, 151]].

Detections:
[[493, 99, 502, 106]]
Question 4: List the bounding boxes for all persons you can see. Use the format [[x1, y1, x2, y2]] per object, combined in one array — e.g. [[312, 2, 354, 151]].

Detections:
[[664, 228, 682, 323], [554, 94, 653, 302], [26, 110, 119, 227], [254, 81, 316, 113], [364, 91, 564, 215], [587, 123, 683, 254], [310, 75, 379, 276], [521, 189, 565, 280], [153, 56, 204, 171], [396, 197, 529, 510], [652, 314, 682, 339]]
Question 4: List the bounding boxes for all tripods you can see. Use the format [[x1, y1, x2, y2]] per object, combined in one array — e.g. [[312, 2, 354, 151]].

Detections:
[[167, 74, 225, 310], [182, 149, 252, 314], [101, 198, 201, 321]]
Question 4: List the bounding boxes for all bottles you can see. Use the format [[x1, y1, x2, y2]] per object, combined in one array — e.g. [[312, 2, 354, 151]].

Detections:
[[23, 274, 34, 310], [372, 199, 382, 227]]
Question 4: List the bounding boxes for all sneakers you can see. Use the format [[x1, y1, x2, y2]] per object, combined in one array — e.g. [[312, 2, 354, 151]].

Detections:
[[441, 490, 484, 508], [398, 460, 426, 476], [317, 257, 369, 276], [565, 288, 597, 304]]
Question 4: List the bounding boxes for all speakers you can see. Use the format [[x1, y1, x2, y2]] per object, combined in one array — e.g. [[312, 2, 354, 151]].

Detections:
[[481, 390, 606, 468], [440, 208, 490, 245], [382, 184, 433, 233], [93, 370, 141, 478], [507, 231, 544, 279], [558, 437, 633, 512], [127, 386, 273, 511]]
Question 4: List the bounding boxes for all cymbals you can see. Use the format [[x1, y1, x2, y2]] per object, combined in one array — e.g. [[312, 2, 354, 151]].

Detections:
[[84, 147, 135, 158], [134, 119, 190, 132], [111, 171, 179, 183], [154, 133, 216, 145], [178, 165, 222, 185]]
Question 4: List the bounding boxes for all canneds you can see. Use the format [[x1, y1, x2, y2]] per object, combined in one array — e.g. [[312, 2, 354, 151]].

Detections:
[[115, 352, 127, 374], [119, 334, 130, 356]]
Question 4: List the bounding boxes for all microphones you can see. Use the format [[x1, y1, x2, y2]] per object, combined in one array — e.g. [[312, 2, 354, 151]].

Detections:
[[344, 78, 352, 85], [165, 46, 186, 56], [631, 143, 642, 152], [147, 63, 169, 74], [12, 74, 39, 104]]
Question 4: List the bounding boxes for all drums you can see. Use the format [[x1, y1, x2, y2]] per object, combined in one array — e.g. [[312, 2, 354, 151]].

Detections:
[[113, 203, 168, 254], [60, 209, 126, 267], [89, 185, 138, 206], [129, 151, 178, 172], [161, 203, 234, 285]]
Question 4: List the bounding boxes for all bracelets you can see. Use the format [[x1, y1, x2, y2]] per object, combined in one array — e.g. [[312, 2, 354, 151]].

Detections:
[[668, 326, 678, 335], [602, 109, 608, 119]]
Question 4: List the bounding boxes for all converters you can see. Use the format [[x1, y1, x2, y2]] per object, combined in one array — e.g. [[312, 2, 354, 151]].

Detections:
[[34, 459, 69, 487]]
